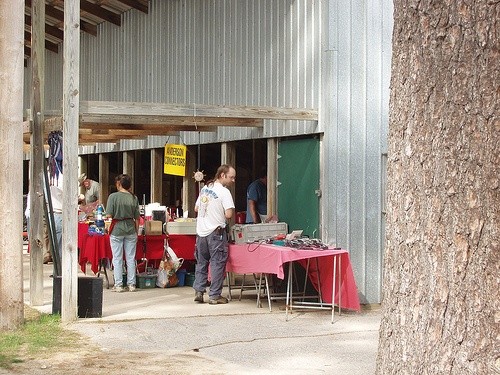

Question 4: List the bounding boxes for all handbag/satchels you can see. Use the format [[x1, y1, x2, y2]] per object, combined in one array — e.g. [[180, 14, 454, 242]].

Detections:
[[159, 239, 184, 279]]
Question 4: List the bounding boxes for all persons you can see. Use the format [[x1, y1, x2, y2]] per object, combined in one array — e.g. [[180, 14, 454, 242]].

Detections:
[[105, 174, 140, 292], [244, 172, 267, 224], [47, 165, 80, 278], [192, 164, 235, 304], [78, 173, 99, 205]]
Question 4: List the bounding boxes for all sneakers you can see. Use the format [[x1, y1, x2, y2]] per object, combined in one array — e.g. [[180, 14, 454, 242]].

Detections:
[[208, 296, 228, 304], [194, 291, 203, 303]]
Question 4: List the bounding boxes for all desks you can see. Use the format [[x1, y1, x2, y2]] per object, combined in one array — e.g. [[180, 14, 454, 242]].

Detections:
[[78, 222, 197, 290], [207, 242, 361, 324]]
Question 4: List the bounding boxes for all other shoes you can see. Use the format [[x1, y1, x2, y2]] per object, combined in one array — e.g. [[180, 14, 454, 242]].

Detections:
[[112, 286, 125, 292], [127, 286, 136, 292], [43, 256, 52, 264]]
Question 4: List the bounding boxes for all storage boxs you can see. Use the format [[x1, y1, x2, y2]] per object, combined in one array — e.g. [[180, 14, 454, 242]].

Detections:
[[133, 273, 158, 289], [147, 221, 162, 236], [185, 273, 196, 287], [177, 269, 186, 286], [113, 272, 128, 288]]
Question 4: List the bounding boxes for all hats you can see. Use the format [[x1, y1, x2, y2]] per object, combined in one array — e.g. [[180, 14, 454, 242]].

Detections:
[[77, 173, 87, 187]]
